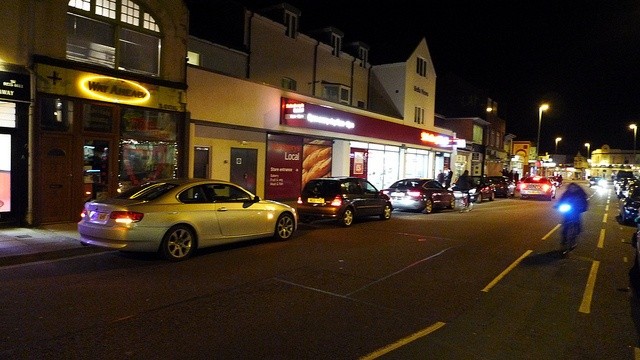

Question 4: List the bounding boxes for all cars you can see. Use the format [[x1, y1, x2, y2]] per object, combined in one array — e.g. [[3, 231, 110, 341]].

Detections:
[[380, 178, 460, 214], [451, 176, 496, 205], [483, 176, 510, 199], [296, 177, 394, 228], [74, 179, 298, 261]]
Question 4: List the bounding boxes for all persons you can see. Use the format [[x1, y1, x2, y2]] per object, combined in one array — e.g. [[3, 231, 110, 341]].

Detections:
[[455, 170, 469, 192], [444, 167, 453, 189], [503, 168, 519, 181], [551, 182, 588, 219]]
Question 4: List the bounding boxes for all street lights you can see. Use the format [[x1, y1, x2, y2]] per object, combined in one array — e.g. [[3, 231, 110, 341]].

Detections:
[[555, 136, 562, 154], [629, 124, 637, 166], [536, 103, 550, 156], [584, 142, 590, 159]]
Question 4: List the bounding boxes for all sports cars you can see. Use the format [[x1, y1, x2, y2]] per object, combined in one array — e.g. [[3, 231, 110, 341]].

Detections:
[[517, 177, 559, 201]]
